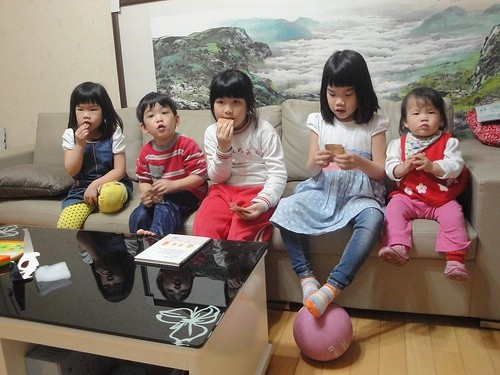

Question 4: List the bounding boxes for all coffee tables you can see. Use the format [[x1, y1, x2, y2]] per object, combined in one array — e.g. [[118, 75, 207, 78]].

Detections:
[[0, 225, 273, 375]]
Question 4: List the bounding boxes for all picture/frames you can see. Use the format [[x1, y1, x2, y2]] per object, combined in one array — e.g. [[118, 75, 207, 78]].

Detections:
[[138, 236, 269, 312], [111, 0, 500, 138]]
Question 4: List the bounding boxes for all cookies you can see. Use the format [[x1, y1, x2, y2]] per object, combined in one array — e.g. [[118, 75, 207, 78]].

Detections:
[[325, 143, 344, 162], [218, 118, 234, 125]]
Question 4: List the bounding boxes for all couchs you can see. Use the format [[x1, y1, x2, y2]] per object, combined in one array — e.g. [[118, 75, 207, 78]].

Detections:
[[0, 138, 500, 329]]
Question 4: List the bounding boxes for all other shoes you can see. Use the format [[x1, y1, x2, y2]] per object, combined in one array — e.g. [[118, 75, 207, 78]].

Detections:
[[378, 245, 410, 266], [443, 260, 470, 281]]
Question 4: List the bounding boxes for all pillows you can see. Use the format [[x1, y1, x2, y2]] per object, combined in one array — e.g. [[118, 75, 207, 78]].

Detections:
[[143, 105, 282, 180], [32, 106, 142, 182], [282, 97, 454, 184], [466, 111, 500, 146], [0, 163, 74, 197]]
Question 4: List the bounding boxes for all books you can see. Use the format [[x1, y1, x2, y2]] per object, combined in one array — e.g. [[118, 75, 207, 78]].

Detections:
[[474, 101, 499, 123], [134, 233, 211, 268]]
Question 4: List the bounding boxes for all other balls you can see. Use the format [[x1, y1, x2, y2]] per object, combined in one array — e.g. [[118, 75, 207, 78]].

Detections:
[[292, 302, 353, 362]]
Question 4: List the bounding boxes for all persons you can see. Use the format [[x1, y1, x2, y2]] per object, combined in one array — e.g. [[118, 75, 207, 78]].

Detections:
[[75, 230, 257, 308], [55, 81, 133, 231], [269, 49, 391, 319], [192, 70, 287, 243], [378, 88, 471, 282], [128, 92, 209, 236]]
